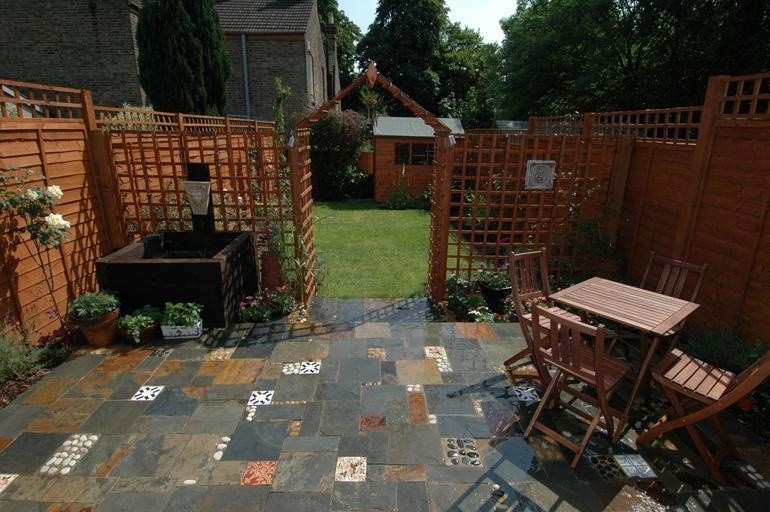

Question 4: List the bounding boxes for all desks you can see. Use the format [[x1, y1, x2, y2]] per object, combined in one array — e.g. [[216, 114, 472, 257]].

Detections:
[[549, 276, 700, 409]]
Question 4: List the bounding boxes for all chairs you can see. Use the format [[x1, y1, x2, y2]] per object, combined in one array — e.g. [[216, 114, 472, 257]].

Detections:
[[501, 246, 593, 391], [636, 346, 770, 484], [606, 250, 708, 407], [524, 300, 630, 469]]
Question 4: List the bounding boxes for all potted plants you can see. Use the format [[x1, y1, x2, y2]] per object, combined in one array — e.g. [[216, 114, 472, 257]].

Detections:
[[471, 269, 510, 312], [160, 301, 204, 339], [67, 288, 119, 346], [116, 307, 160, 342]]
